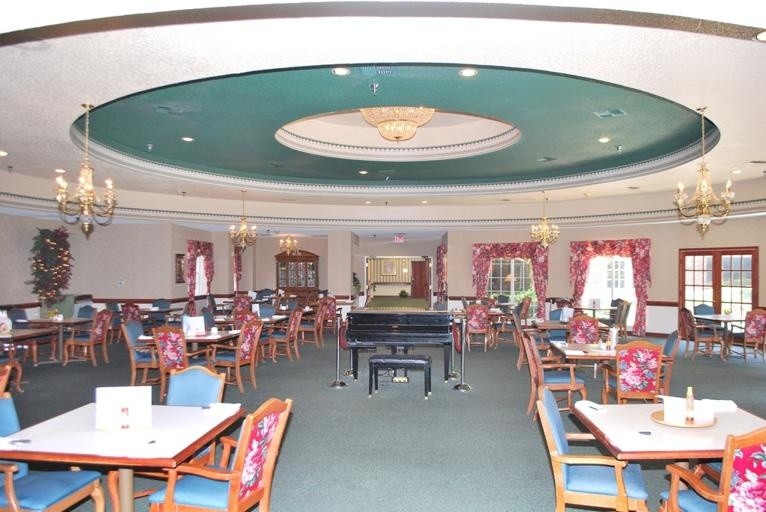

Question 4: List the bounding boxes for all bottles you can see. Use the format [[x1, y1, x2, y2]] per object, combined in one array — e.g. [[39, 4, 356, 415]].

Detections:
[[685, 386, 696, 424], [120, 406, 128, 428], [605, 335, 611, 350], [54, 308, 58, 321]]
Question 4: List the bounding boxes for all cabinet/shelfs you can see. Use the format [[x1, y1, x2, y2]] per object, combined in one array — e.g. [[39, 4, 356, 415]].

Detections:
[[274, 249, 320, 321]]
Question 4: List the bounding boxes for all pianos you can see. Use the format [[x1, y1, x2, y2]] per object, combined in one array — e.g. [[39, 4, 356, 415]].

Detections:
[[347, 311, 453, 383]]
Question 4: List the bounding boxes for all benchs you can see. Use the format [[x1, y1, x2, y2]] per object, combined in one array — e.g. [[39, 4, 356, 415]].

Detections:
[[368, 354, 432, 401]]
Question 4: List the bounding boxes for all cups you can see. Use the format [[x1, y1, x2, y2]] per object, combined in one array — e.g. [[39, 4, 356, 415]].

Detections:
[[210, 326, 218, 337]]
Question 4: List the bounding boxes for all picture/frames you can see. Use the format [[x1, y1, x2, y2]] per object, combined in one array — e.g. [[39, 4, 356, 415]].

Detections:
[[380, 259, 397, 276]]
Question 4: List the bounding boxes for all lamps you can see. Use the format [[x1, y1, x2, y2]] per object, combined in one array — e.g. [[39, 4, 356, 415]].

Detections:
[[358, 107, 436, 145], [527, 197, 560, 250], [54, 103, 118, 235], [277, 234, 300, 258], [226, 189, 257, 249], [673, 106, 736, 235]]
